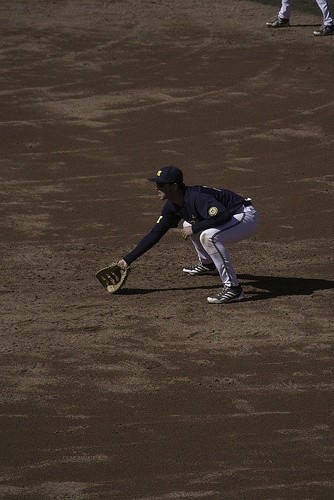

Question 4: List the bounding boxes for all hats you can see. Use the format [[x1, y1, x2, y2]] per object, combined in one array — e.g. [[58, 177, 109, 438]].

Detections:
[[149, 167, 183, 184]]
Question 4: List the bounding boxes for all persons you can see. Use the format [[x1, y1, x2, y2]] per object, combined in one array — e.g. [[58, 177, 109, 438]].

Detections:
[[265, 0, 334, 36], [119, 165, 257, 304]]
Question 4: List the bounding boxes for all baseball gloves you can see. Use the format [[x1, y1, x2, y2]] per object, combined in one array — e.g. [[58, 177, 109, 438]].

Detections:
[[96, 261, 129, 294]]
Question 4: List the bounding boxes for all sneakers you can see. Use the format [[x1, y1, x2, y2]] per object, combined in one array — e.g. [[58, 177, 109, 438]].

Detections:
[[183, 264, 220, 276], [266, 16, 289, 27], [207, 284, 244, 304], [313, 24, 334, 36]]
